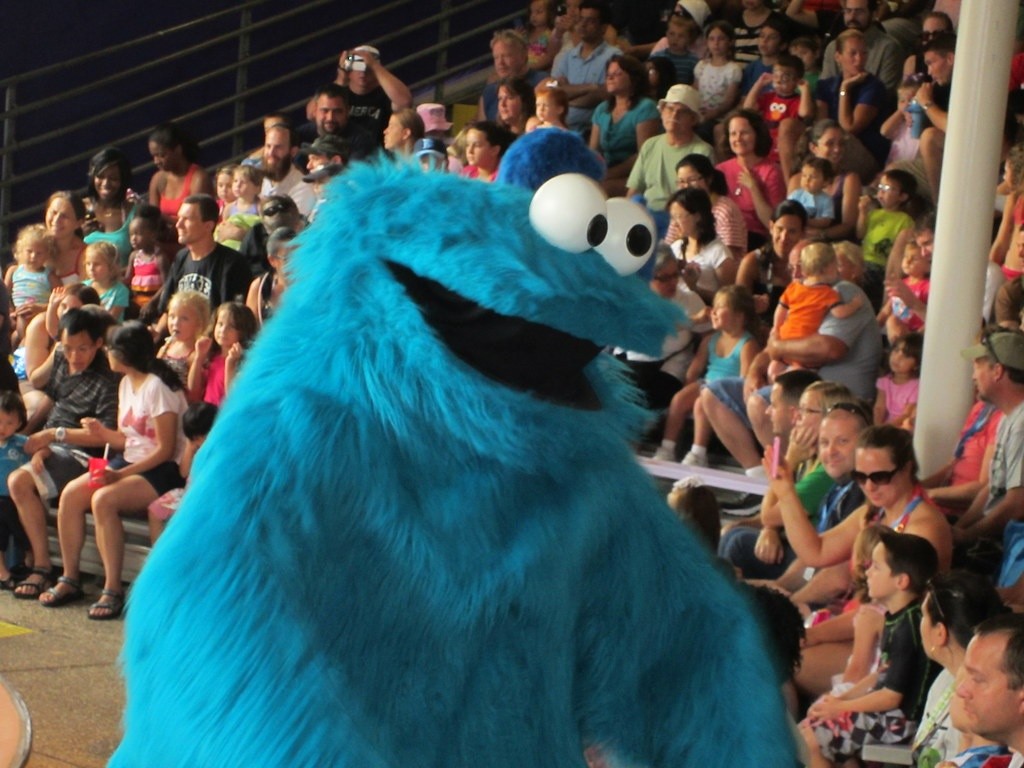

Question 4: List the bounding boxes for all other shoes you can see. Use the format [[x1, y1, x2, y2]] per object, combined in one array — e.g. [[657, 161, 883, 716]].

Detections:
[[8, 561, 32, 580], [680, 451, 709, 469], [1, 572, 20, 591], [651, 447, 676, 464]]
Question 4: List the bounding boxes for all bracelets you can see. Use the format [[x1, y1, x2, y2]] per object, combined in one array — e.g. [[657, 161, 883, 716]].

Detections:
[[839, 89, 848, 96], [546, 78, 559, 88], [923, 101, 935, 111]]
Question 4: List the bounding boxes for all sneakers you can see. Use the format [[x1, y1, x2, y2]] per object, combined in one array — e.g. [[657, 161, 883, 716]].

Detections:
[[719, 493, 765, 517]]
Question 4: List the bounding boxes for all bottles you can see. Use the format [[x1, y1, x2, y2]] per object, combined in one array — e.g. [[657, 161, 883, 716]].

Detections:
[[891, 294, 925, 331]]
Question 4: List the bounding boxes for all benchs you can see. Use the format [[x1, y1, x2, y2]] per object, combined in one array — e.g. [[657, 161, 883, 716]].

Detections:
[[43, 505, 151, 582]]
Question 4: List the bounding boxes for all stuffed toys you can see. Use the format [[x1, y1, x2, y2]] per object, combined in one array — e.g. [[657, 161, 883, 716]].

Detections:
[[498, 126, 669, 288], [103, 156, 798, 768]]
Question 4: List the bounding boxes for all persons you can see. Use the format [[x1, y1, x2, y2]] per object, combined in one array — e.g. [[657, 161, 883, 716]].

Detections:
[[781, 427, 951, 724], [39, 321, 188, 621], [0, 49, 453, 383], [795, 534, 938, 768], [12, 284, 100, 433], [186, 304, 256, 405], [47, 287, 103, 345], [148, 401, 218, 544], [603, 145, 1024, 683], [0, 392, 31, 590], [444, 0, 1024, 243], [0, 311, 118, 599], [912, 572, 1011, 768], [938, 610, 1024, 768]]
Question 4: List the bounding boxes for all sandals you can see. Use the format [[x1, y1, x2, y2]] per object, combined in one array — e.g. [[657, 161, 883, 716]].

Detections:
[[38, 576, 84, 607], [13, 567, 57, 599], [88, 589, 125, 620]]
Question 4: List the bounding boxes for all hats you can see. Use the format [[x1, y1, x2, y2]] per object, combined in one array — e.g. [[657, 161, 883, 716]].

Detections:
[[416, 103, 453, 133], [656, 84, 705, 124], [301, 135, 353, 159], [303, 163, 343, 183], [678, 0, 712, 29], [351, 45, 381, 74], [413, 138, 447, 162], [960, 332, 1023, 373]]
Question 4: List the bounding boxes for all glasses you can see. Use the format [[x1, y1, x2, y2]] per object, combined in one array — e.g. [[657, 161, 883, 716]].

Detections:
[[653, 271, 679, 282], [263, 203, 288, 217], [925, 572, 946, 624], [675, 175, 704, 187], [980, 334, 1001, 364], [673, 11, 686, 19], [877, 183, 902, 193], [918, 30, 945, 42], [794, 406, 825, 420], [850, 457, 906, 485]]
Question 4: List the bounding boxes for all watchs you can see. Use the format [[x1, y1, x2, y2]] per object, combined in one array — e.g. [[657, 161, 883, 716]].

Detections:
[[55, 426, 66, 443]]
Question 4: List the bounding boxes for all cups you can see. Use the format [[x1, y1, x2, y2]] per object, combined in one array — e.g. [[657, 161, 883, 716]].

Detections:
[[88, 457, 108, 489], [912, 100, 928, 138]]
